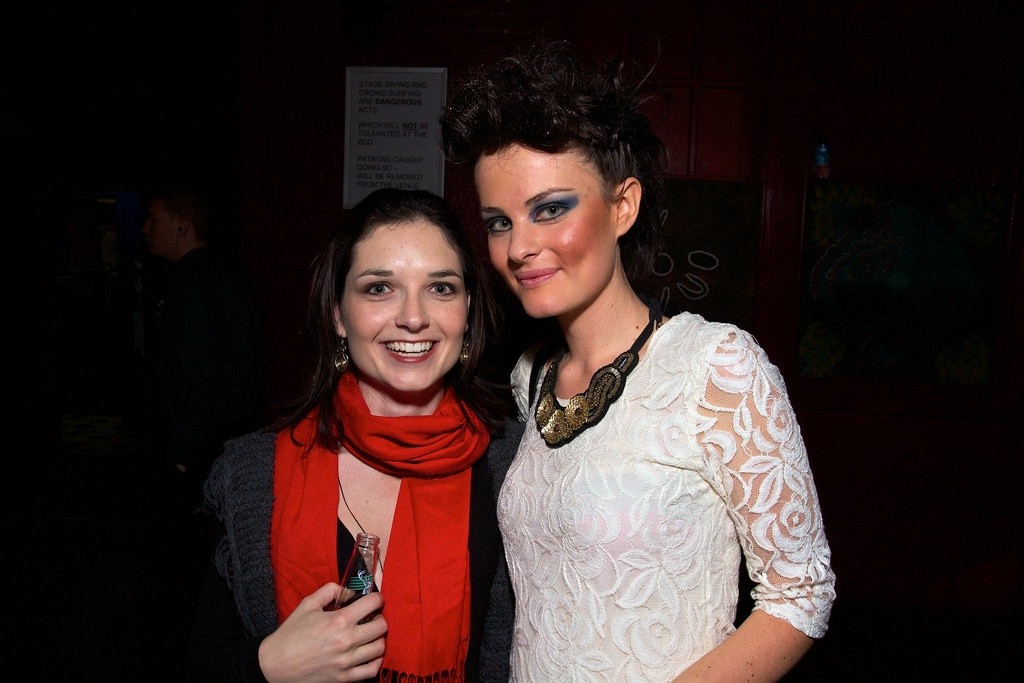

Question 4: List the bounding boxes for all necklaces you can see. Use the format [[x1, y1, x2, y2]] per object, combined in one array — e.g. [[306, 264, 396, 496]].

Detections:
[[337, 477, 382, 572], [534, 307, 661, 448]]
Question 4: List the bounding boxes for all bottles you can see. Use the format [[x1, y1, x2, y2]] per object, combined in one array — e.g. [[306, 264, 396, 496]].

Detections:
[[808, 132, 838, 256], [327, 533, 381, 625]]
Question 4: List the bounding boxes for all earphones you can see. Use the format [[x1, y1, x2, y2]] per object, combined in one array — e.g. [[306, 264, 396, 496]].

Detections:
[[178, 227, 182, 232]]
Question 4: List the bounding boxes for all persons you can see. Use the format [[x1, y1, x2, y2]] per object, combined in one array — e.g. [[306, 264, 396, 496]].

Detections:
[[440, 44, 837, 683], [139, 187, 264, 482], [186, 189, 526, 683]]
